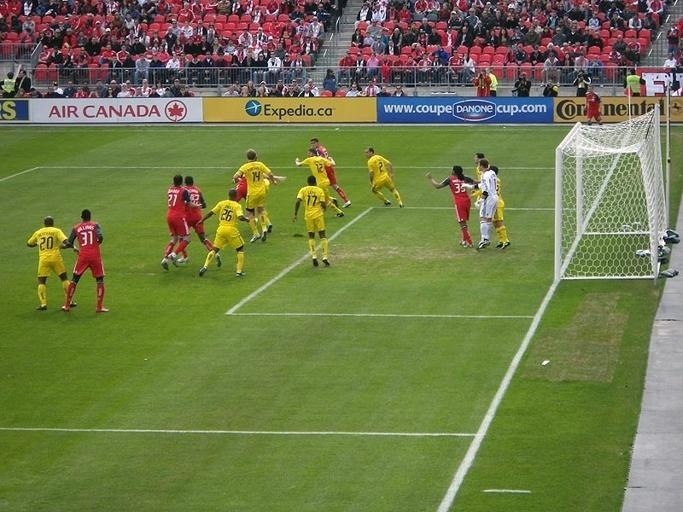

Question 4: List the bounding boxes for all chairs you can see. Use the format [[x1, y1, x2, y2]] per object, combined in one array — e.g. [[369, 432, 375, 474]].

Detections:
[[0, 1, 682, 89]]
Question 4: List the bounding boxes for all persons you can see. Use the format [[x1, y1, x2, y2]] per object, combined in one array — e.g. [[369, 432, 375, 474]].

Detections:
[[160, 174, 191, 272], [338, 1, 683, 100], [194, 188, 250, 277], [291, 174, 331, 267], [173, 175, 222, 268], [309, 137, 351, 209], [582, 90, 606, 131], [1, 1, 346, 98], [231, 146, 511, 254], [24, 216, 78, 310], [59, 208, 110, 314]]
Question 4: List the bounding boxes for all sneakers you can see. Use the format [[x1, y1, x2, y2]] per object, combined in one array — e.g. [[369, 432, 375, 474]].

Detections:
[[36, 306, 47, 313], [312, 256, 319, 266], [198, 253, 246, 276], [322, 257, 330, 267], [332, 198, 351, 217], [400, 204, 404, 208], [62, 305, 69, 312], [161, 252, 189, 271], [96, 307, 109, 312], [384, 201, 391, 207], [250, 224, 272, 243], [459, 238, 510, 250], [71, 301, 77, 307]]
